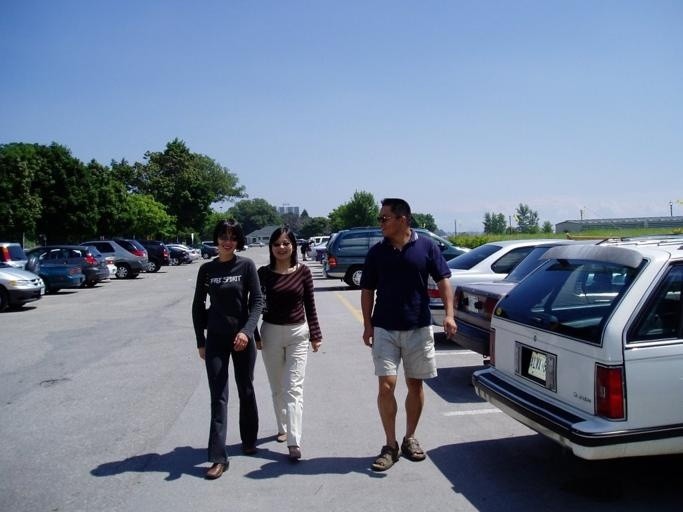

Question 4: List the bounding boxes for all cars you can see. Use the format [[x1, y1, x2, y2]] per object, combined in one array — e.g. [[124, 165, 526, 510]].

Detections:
[[0, 262, 46, 309], [316, 247, 327, 264], [427, 239, 574, 326], [164, 240, 219, 265], [35, 262, 85, 294], [78, 239, 148, 278], [295, 239, 306, 246], [311, 240, 330, 262], [447, 237, 683, 358], [248, 242, 265, 248]]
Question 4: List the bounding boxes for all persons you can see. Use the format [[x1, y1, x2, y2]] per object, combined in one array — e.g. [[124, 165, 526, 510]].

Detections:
[[249, 225, 324, 463], [190, 216, 262, 478], [300, 238, 314, 261], [358, 196, 457, 473]]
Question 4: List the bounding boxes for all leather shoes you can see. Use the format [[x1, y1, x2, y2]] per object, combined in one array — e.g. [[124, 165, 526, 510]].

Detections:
[[277, 434, 287, 441], [207, 463, 228, 478], [289, 447, 300, 458], [243, 442, 256, 454]]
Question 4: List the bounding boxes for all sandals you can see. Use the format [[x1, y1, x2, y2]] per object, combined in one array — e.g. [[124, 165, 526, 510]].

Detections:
[[372, 443, 399, 471], [401, 437, 425, 460]]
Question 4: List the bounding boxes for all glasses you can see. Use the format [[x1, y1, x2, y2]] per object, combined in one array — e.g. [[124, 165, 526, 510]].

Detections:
[[219, 235, 235, 241], [377, 215, 401, 221], [272, 240, 290, 246]]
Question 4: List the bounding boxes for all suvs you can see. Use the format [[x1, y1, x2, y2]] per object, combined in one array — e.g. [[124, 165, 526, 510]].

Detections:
[[138, 240, 170, 272], [26, 246, 109, 288], [325, 227, 472, 289], [304, 236, 330, 261], [0, 242, 39, 274], [471, 238, 683, 460]]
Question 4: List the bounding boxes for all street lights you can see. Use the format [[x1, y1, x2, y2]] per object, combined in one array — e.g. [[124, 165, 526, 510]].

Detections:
[[578, 208, 584, 220], [219, 206, 222, 211], [423, 222, 426, 229], [507, 215, 512, 233], [454, 219, 457, 235], [669, 200, 673, 215]]
